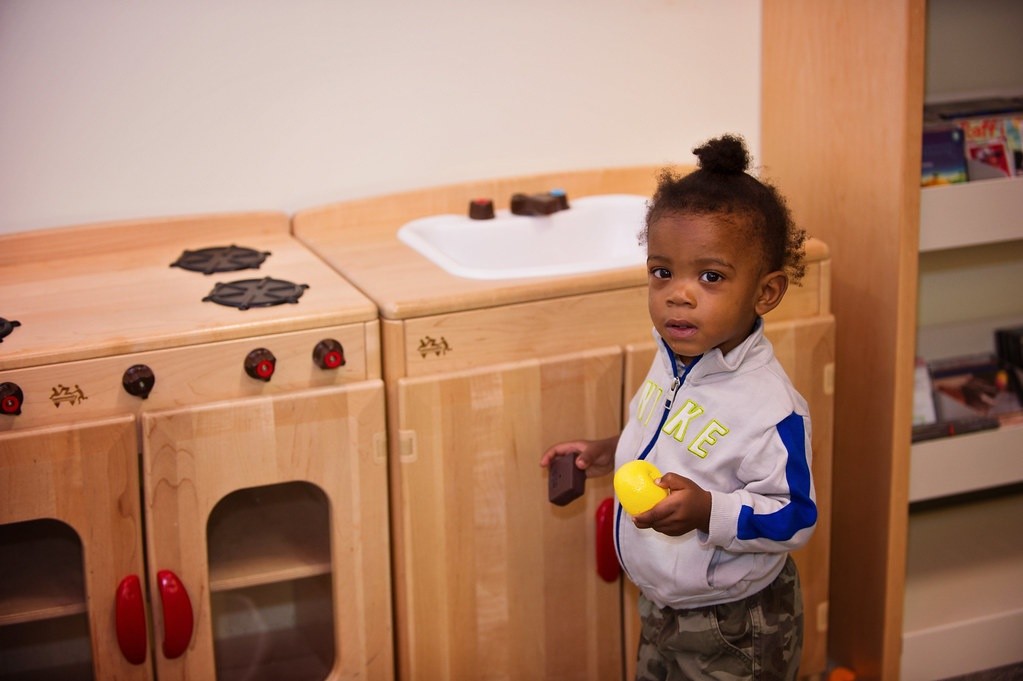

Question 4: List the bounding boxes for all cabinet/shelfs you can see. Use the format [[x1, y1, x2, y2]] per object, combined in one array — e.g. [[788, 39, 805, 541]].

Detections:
[[393, 315, 837, 681], [759, 0, 1023, 681], [0, 380, 394, 681]]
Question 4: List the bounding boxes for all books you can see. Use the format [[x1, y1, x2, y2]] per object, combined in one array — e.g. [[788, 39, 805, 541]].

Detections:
[[919, 112, 1023, 188]]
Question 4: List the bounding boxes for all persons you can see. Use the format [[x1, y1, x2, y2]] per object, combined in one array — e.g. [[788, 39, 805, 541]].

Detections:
[[539, 133, 818, 681]]
